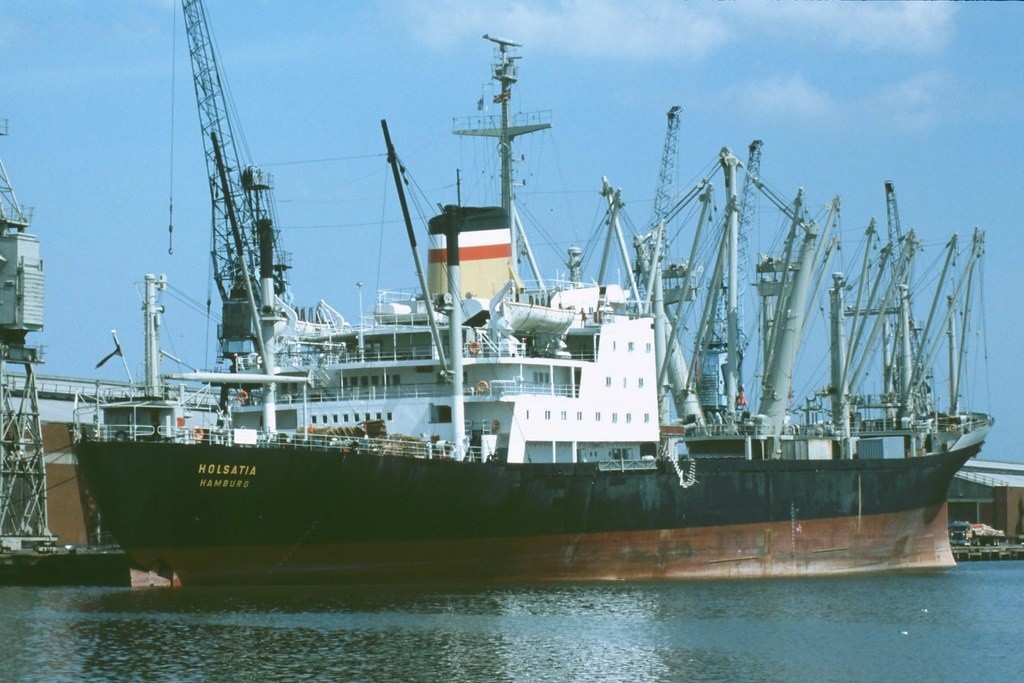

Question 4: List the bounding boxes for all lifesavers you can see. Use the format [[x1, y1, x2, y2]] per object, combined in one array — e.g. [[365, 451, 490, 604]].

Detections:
[[237, 391, 248, 402], [469, 342, 480, 354], [115, 430, 127, 443], [477, 381, 489, 393], [192, 427, 204, 441]]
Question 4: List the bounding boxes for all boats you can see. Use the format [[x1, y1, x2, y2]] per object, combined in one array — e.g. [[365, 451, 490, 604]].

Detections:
[[69, 117, 989, 592]]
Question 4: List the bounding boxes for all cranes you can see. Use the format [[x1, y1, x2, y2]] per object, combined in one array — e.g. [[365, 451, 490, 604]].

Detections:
[[451, 33, 995, 459], [0, 116, 133, 557], [167, 0, 297, 374]]
